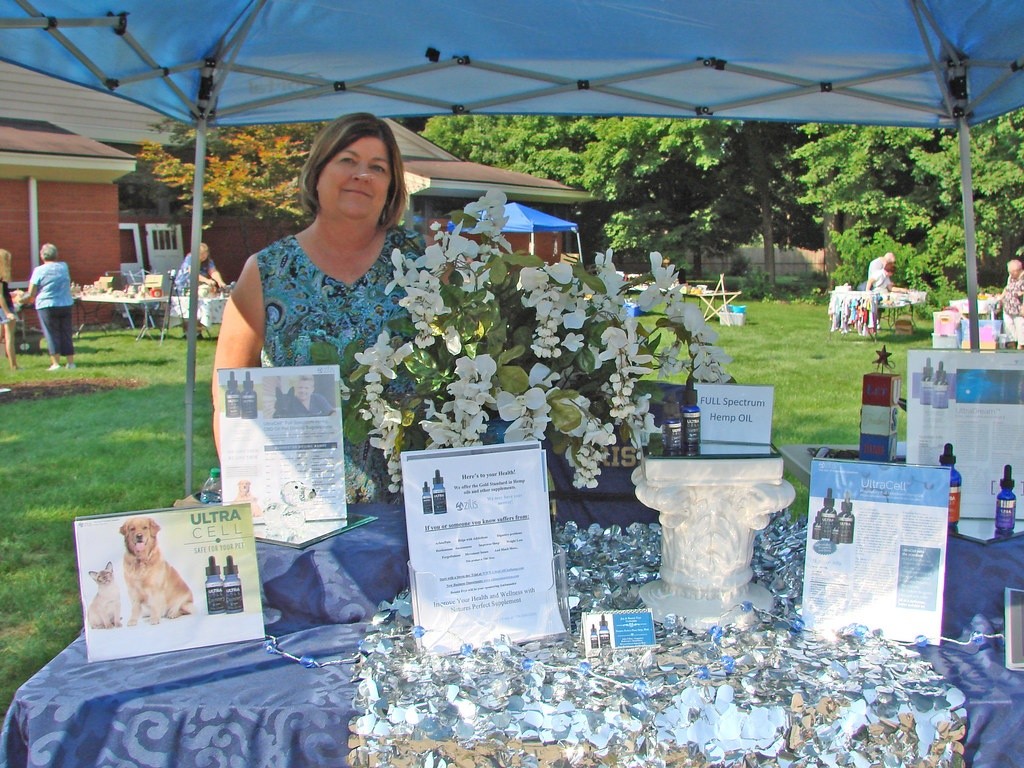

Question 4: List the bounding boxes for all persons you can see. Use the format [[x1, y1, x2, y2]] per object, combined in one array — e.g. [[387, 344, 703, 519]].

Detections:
[[996, 259, 1024, 350], [866, 263, 896, 330], [211, 111, 429, 503], [590, 614, 610, 648], [20, 243, 75, 370], [274, 372, 335, 418], [868, 252, 895, 279], [812, 487, 854, 544], [920, 357, 950, 408], [0, 249, 20, 369], [176, 243, 225, 340]]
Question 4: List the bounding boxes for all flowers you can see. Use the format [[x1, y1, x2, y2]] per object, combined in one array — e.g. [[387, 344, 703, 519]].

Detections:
[[309, 186, 738, 487]]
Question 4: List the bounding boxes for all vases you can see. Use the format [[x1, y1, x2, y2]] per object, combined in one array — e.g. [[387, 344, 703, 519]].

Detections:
[[478, 415, 515, 446]]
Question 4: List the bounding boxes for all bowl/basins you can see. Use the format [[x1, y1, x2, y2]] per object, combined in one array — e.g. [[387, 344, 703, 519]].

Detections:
[[721, 305, 732, 311], [733, 306, 746, 313]]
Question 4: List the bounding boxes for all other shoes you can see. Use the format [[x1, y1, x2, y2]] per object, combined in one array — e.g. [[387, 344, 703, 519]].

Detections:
[[67, 363, 76, 368], [46, 364, 61, 371]]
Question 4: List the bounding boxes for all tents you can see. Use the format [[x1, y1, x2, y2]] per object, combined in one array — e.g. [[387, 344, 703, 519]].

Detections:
[[447, 202, 583, 264]]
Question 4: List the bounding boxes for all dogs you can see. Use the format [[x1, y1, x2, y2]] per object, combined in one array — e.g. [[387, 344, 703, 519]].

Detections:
[[119, 517, 195, 627]]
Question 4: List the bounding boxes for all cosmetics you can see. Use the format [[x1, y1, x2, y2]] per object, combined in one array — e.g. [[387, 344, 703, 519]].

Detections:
[[205, 554, 244, 615], [939, 443, 962, 526], [994, 463, 1017, 531], [422, 469, 447, 514], [225, 370, 257, 419]]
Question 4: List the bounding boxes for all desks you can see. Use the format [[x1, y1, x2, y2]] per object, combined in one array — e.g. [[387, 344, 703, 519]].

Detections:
[[0, 442, 1024, 768], [626, 288, 728, 314], [949, 294, 998, 320], [167, 295, 229, 338], [878, 298, 928, 332], [71, 296, 169, 341]]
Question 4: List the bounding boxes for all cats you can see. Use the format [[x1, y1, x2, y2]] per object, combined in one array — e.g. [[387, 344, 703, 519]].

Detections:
[[87, 560, 123, 629]]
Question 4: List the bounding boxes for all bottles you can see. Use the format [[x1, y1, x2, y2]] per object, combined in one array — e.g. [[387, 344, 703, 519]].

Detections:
[[939, 443, 962, 529], [240, 371, 257, 419], [421, 481, 433, 514], [204, 555, 225, 615], [598, 614, 612, 649], [590, 623, 599, 648], [919, 357, 948, 409], [225, 371, 240, 418], [200, 467, 222, 504], [223, 555, 244, 613], [995, 465, 1017, 534], [813, 487, 855, 545], [661, 379, 702, 452], [432, 469, 447, 514]]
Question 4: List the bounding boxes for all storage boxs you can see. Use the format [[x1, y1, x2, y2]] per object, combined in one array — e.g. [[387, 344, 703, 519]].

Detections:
[[719, 312, 746, 325], [961, 320, 1003, 341], [621, 299, 645, 317], [931, 333, 962, 348], [934, 310, 962, 335]]
